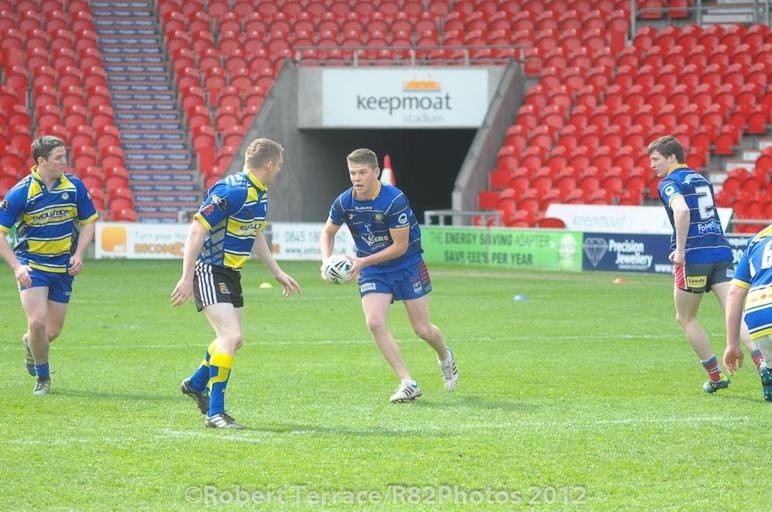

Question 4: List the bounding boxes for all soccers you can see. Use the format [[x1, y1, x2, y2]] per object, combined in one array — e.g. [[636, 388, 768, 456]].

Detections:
[[322, 255, 352, 284]]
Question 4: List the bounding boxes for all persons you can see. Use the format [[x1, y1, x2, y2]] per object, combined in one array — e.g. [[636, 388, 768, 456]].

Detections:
[[170, 137, 302, 429], [647, 135, 772, 402], [319, 149, 457, 402], [722, 223, 772, 372], [0, 134, 99, 396]]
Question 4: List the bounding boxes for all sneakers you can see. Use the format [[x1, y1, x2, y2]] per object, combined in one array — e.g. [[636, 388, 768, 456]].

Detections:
[[390, 380, 422, 401], [33, 375, 51, 395], [437, 347, 459, 392], [204, 413, 248, 430], [701, 377, 733, 393], [22, 332, 38, 377], [181, 377, 211, 414], [759, 365, 772, 402]]
